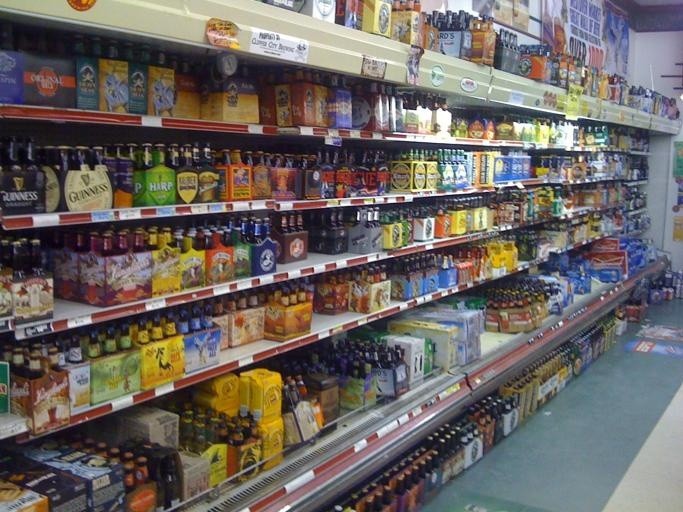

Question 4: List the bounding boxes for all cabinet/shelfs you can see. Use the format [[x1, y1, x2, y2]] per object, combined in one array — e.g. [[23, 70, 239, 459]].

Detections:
[[1, 0, 476, 512], [412, 47, 681, 444]]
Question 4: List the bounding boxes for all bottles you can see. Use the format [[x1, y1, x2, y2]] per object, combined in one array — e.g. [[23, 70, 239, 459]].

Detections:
[[450, 106, 611, 132], [0, 147, 466, 171], [540, 149, 652, 237], [392, 0, 652, 96], [332, 313, 619, 511], [1, 282, 315, 379], [0, 190, 539, 279], [469, 280, 561, 308], [1, 23, 450, 112], [68, 335, 406, 496]]
